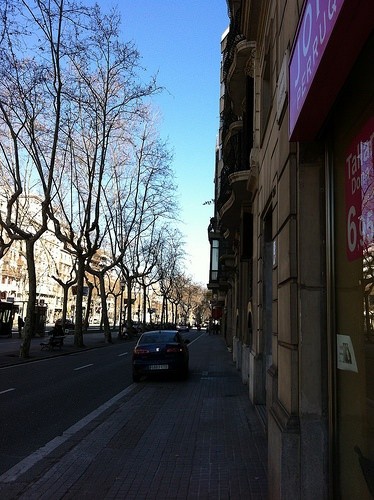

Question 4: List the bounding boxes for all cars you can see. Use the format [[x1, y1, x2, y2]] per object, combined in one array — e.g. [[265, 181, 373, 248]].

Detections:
[[132, 330, 190, 382], [177, 323, 189, 332]]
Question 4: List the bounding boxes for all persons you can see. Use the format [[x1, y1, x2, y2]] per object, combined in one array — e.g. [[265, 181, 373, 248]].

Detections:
[[7, 318, 13, 338], [120, 325, 128, 339], [18, 316, 23, 338], [207, 323, 219, 335], [48, 325, 65, 343], [197, 323, 201, 328]]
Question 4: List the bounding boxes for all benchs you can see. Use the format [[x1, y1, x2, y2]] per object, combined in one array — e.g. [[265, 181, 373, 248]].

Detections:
[[40, 336, 65, 351]]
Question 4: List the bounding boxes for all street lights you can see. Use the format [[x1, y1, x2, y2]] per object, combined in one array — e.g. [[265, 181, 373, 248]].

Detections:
[[118, 277, 126, 342]]
